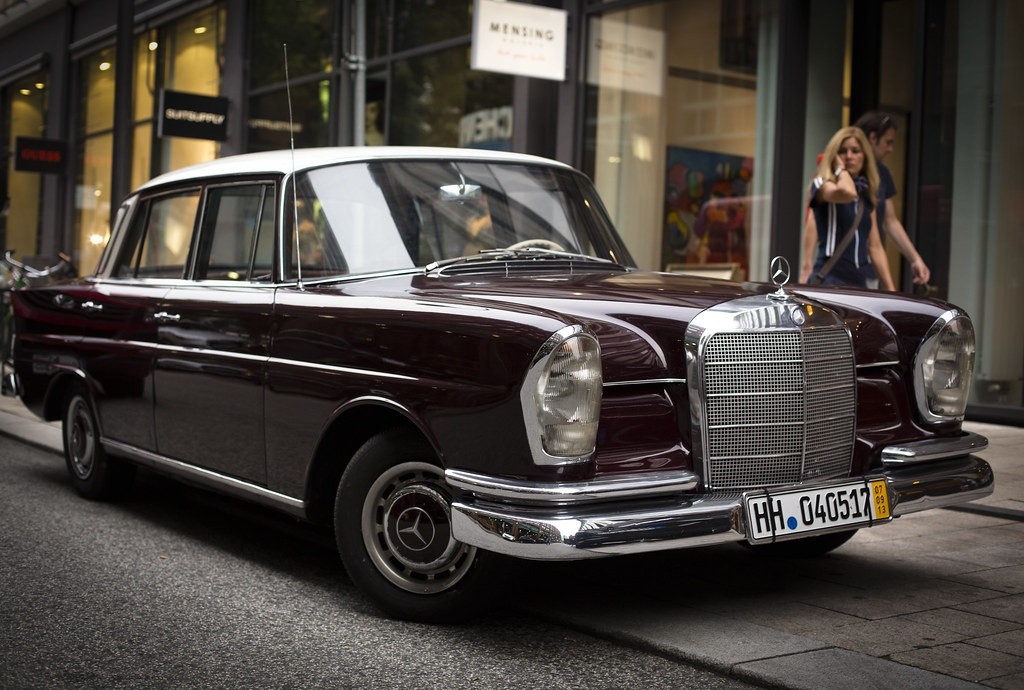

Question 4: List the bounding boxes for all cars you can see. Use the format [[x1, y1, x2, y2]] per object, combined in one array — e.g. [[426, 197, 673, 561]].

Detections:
[[10, 144, 997, 619]]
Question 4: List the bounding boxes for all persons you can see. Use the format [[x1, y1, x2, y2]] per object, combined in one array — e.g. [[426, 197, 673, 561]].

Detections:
[[293, 199, 325, 267], [462, 188, 496, 255], [798, 110, 930, 291]]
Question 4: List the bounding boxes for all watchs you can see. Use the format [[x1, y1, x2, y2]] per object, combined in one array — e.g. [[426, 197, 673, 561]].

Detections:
[[834, 167, 846, 181]]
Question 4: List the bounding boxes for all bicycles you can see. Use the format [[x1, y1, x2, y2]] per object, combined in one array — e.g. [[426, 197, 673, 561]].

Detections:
[[0, 248, 75, 396]]
